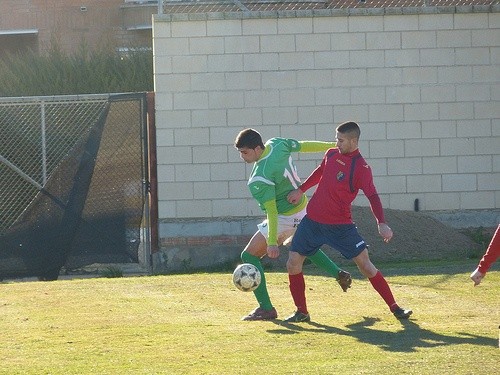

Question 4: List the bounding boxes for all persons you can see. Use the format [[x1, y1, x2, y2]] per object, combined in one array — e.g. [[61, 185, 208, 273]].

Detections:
[[285, 121, 413, 322], [234, 128, 352, 322], [471, 224, 500, 287]]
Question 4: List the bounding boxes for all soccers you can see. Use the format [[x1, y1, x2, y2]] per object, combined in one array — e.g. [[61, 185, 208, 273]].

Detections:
[[232, 263, 262, 293]]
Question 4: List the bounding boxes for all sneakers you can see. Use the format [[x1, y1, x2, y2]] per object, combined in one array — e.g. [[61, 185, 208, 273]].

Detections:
[[336, 270, 352, 292], [471, 269, 485, 284], [243, 307, 277, 320], [285, 311, 310, 322], [393, 307, 412, 319]]
